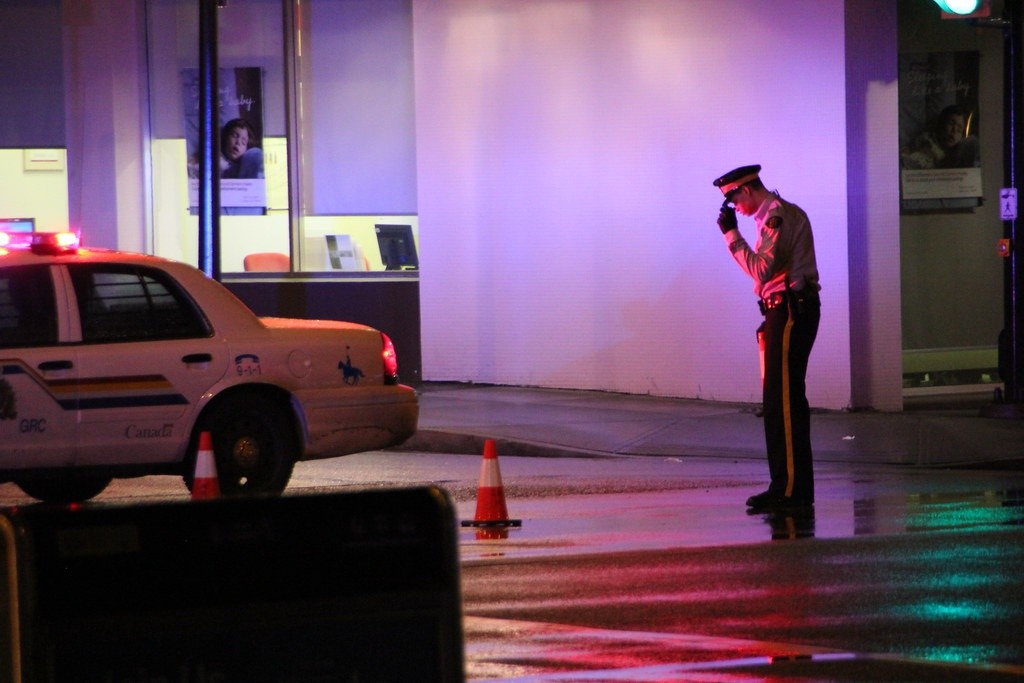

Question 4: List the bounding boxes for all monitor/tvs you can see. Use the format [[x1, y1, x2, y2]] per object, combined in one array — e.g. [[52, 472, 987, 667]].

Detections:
[[374, 225, 419, 270], [0, 218, 35, 233]]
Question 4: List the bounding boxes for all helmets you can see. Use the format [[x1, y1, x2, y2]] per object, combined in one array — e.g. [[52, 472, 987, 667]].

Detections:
[[713, 164, 761, 206]]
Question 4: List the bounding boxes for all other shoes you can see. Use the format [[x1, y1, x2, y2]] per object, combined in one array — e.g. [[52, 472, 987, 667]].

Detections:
[[767, 500, 812, 514], [746, 491, 779, 506]]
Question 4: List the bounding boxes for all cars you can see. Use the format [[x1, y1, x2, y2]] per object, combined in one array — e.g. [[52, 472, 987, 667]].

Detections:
[[0, 228, 419, 505]]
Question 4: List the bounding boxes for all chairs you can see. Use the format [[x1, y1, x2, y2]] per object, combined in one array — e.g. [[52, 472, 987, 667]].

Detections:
[[244, 252, 292, 273]]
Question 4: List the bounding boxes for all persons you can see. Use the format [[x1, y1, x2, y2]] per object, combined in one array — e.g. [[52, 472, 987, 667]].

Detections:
[[188, 116, 264, 179], [901, 108, 982, 170], [712, 165, 822, 509]]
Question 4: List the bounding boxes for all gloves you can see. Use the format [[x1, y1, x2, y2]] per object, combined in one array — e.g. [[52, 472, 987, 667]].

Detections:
[[717, 206, 737, 235], [756, 321, 765, 344]]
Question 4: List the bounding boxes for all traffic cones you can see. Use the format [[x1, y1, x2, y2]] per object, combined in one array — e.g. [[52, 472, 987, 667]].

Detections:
[[188, 431, 221, 503], [457, 438, 523, 529]]
